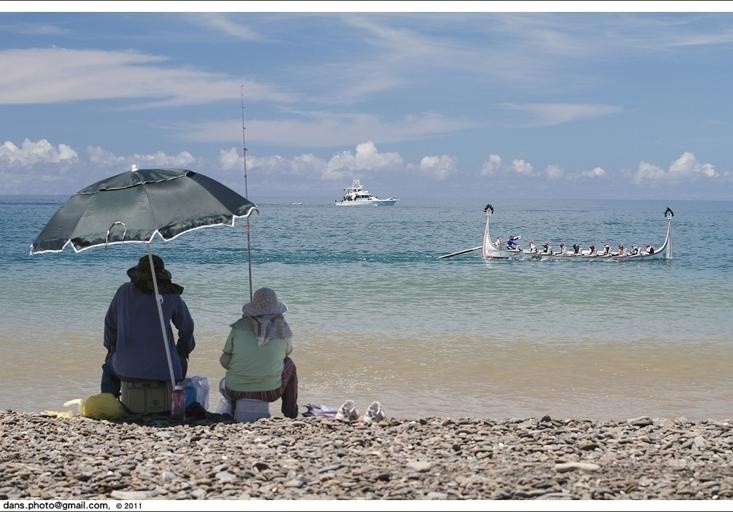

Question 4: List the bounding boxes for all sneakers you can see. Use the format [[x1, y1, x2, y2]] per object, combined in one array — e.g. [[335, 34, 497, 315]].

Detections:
[[364, 401, 385, 421], [337, 401, 360, 423]]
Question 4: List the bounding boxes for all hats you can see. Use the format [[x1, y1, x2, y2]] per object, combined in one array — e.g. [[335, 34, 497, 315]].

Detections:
[[126, 254, 172, 283], [240, 286, 289, 317]]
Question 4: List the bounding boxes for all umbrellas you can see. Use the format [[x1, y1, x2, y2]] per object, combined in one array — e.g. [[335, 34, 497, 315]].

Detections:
[[30, 162, 259, 391]]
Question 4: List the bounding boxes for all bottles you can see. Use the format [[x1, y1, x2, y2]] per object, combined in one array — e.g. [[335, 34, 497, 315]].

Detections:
[[171, 386, 185, 420]]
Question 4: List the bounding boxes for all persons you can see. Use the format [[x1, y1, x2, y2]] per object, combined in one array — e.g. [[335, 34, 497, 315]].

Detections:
[[100, 253, 195, 399], [216, 288, 299, 418], [493, 235, 655, 259]]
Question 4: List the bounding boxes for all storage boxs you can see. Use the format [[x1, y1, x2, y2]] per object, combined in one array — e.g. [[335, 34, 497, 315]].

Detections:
[[119, 379, 174, 416], [232, 398, 270, 424]]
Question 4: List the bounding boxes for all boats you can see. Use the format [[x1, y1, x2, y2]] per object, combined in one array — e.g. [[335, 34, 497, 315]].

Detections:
[[481, 201, 675, 263], [333, 177, 400, 208], [292, 202, 302, 206]]
